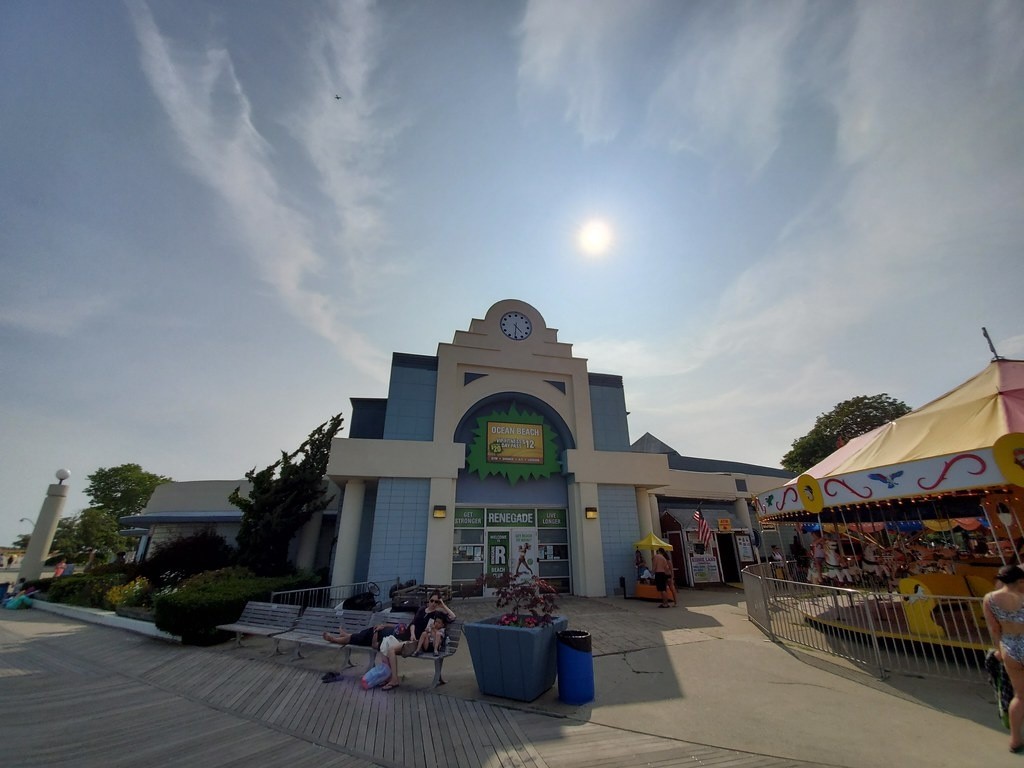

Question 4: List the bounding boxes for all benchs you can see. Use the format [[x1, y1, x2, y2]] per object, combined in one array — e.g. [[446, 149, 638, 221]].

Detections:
[[273, 607, 374, 673], [216, 601, 301, 657], [345, 611, 463, 691]]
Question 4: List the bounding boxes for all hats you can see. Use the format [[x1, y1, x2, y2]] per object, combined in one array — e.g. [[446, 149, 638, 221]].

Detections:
[[394, 623, 407, 636]]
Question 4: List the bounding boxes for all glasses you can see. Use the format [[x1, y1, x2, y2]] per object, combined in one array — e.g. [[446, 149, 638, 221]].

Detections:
[[774, 547, 780, 550], [429, 598, 440, 603]]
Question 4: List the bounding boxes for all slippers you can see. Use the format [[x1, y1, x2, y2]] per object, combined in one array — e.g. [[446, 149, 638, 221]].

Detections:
[[322, 670, 342, 679], [1008, 738, 1024, 753], [381, 680, 400, 690], [323, 675, 345, 684]]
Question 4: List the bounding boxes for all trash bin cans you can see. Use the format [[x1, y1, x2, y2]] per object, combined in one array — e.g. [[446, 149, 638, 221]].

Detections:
[[556, 629, 596, 705], [0, 582, 11, 604]]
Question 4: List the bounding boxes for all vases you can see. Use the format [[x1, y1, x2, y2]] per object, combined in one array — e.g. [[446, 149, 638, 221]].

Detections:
[[464, 612, 568, 702]]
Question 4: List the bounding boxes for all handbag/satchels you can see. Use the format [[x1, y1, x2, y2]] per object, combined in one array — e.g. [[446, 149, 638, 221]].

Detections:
[[401, 639, 419, 658]]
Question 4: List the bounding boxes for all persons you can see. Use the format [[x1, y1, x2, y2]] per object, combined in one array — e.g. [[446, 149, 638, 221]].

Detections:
[[651, 548, 679, 609], [633, 549, 647, 569], [381, 589, 456, 691], [769, 544, 789, 581], [513, 542, 533, 578], [982, 566, 1024, 754], [322, 623, 408, 650], [791, 531, 1024, 583], [0, 551, 126, 611], [410, 612, 447, 657]]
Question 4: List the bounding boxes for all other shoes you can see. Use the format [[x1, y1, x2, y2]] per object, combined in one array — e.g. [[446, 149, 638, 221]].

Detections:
[[432, 648, 440, 657], [411, 650, 419, 657], [658, 603, 671, 609], [672, 601, 680, 607]]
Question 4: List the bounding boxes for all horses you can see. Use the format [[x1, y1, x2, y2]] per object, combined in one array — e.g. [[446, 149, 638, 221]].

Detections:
[[806, 537, 957, 594]]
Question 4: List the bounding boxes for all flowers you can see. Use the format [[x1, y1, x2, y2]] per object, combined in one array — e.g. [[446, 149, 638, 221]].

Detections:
[[459, 570, 562, 631]]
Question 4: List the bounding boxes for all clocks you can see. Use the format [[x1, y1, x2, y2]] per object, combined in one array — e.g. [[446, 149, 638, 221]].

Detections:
[[500, 311, 532, 341]]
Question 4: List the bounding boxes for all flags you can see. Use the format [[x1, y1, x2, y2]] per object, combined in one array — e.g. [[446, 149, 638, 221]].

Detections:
[[693, 505, 714, 550]]
